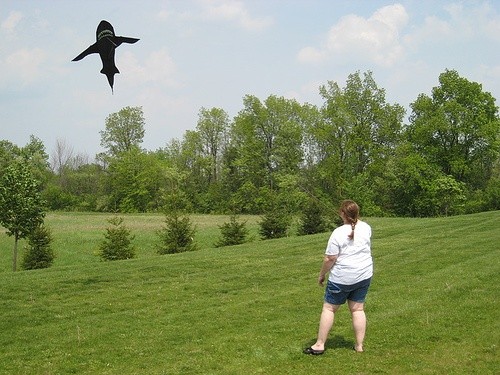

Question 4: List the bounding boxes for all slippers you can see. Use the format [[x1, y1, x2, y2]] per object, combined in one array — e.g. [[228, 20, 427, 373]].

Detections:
[[303, 347, 324, 355], [352, 344, 365, 353]]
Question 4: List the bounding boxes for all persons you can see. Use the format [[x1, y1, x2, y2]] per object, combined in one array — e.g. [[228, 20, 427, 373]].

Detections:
[[304, 200, 374, 355]]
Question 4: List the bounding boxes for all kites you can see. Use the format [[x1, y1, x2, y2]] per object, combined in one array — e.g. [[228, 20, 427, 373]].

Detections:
[[70, 20, 140, 93]]
